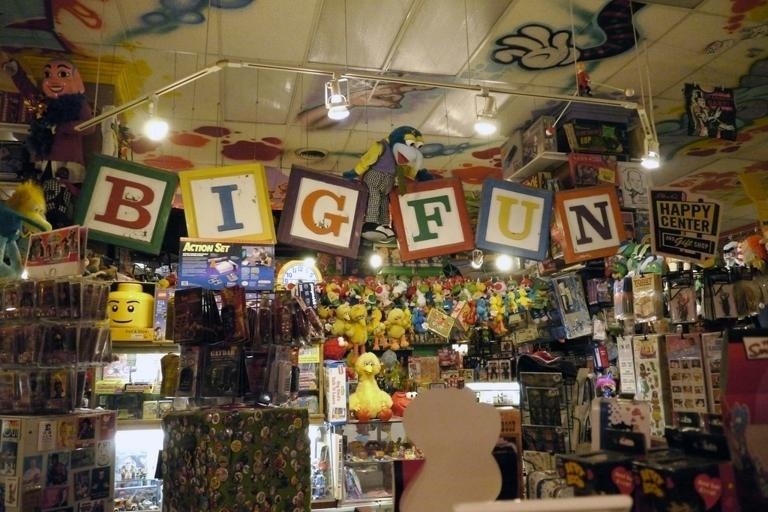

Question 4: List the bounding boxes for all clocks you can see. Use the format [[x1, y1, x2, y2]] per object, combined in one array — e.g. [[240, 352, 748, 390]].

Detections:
[[277, 261, 322, 287]]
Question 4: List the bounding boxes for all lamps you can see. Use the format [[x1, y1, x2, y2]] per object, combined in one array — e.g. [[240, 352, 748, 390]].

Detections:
[[75, 60, 661, 169]]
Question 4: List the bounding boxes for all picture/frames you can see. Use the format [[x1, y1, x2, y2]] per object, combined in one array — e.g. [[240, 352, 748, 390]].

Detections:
[[476, 178, 554, 261], [556, 184, 627, 265], [278, 164, 368, 260], [389, 177, 474, 262], [179, 163, 277, 243], [73, 154, 179, 256]]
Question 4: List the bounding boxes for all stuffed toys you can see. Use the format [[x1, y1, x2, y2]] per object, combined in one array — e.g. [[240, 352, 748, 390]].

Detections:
[[343, 125, 424, 244], [604, 238, 665, 279], [317, 275, 551, 422], [722, 225, 768, 271]]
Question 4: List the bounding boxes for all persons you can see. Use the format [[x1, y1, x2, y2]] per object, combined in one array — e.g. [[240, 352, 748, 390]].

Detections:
[[3, 57, 93, 192], [550, 337, 596, 401]]
